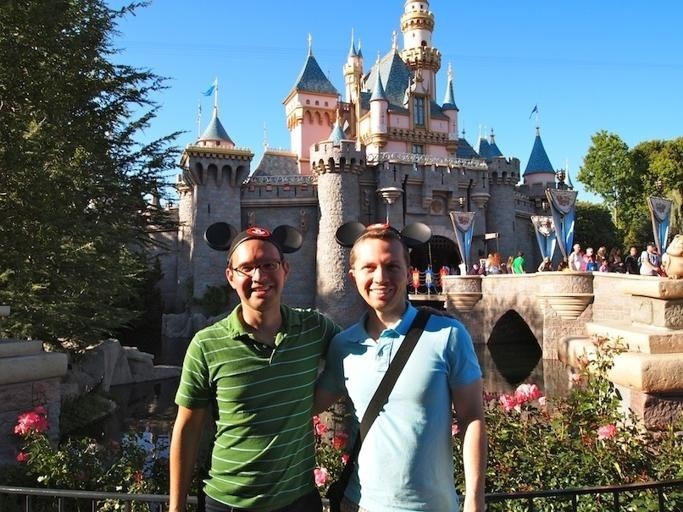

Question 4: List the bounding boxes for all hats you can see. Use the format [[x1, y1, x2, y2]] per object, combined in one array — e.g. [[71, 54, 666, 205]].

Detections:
[[204, 221, 303, 263], [335, 220, 433, 250]]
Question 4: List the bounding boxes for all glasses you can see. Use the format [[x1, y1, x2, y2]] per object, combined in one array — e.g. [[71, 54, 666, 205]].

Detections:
[[233, 260, 280, 276]]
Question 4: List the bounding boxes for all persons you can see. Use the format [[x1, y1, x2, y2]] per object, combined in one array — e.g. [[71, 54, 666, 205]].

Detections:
[[468, 251, 527, 276], [537, 255, 552, 273], [313, 223, 489, 512], [168, 227, 343, 512], [559, 241, 667, 277]]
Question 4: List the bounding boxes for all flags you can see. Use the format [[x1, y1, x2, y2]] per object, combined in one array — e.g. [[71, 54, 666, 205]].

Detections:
[[529, 106, 539, 119], [200, 81, 216, 97]]
